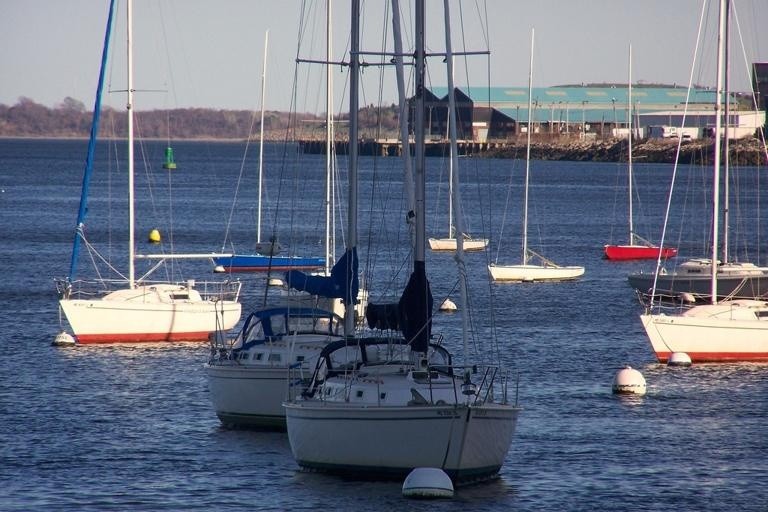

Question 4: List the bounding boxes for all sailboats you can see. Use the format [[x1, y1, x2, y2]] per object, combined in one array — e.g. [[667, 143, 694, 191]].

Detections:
[[428, 142, 492, 250], [277, 0, 531, 501], [485, 30, 587, 284], [602, 1, 768, 367], [50, 1, 247, 348], [213, 29, 326, 273], [205, 0, 385, 433]]
